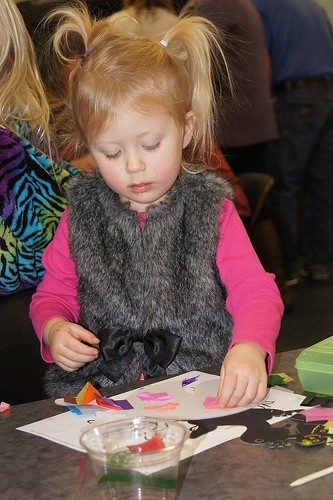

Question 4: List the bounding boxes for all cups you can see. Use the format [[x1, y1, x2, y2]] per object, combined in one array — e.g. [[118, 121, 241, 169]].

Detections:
[[79, 416, 189, 500]]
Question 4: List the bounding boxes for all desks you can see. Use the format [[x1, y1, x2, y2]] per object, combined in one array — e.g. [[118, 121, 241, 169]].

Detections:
[[0, 350, 333, 500]]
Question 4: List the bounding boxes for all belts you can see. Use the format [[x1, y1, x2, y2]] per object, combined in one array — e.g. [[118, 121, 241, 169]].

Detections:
[[273, 74, 333, 92]]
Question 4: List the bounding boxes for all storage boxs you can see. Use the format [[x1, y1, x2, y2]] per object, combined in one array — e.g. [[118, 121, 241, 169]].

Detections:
[[294, 336, 333, 397]]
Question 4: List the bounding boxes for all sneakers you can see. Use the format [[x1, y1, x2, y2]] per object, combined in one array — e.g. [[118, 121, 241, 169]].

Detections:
[[300, 266, 331, 281], [284, 267, 300, 286]]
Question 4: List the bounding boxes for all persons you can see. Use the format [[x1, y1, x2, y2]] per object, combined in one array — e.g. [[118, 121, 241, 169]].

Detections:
[[29, 1, 286, 408], [1, 0, 82, 412], [254, 4, 333, 286], [69, 0, 292, 311]]
